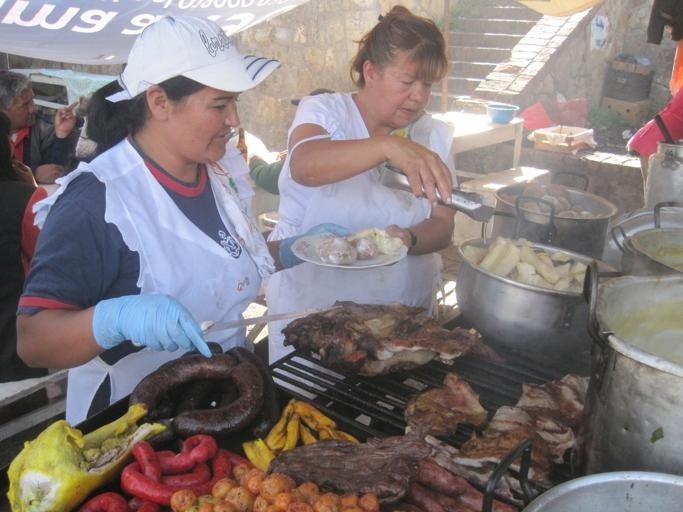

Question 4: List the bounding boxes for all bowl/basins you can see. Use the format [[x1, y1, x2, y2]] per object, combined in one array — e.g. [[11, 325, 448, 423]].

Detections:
[[485, 102, 519, 123]]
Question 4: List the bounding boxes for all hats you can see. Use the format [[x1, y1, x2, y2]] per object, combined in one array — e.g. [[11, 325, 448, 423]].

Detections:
[[103, 14, 283, 105]]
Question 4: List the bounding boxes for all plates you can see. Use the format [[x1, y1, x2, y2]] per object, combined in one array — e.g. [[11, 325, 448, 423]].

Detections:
[[290, 234, 410, 272]]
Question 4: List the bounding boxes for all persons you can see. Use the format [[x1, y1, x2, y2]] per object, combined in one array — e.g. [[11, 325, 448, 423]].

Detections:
[[267, 4, 461, 367], [0, 66, 82, 188], [75, 72, 131, 170], [245, 88, 344, 196], [16, 13, 354, 434], [0, 111, 53, 383]]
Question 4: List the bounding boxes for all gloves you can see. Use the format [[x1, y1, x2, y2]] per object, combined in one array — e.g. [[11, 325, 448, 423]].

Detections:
[[92, 291, 213, 359], [277, 221, 352, 271]]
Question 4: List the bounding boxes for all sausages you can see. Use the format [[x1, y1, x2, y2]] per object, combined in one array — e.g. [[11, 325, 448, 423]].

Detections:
[[77, 342, 277, 511], [392, 458, 517, 512]]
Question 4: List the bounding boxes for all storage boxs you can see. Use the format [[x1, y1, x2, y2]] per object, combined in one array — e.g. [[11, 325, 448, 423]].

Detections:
[[605, 61, 655, 100], [602, 98, 651, 125]]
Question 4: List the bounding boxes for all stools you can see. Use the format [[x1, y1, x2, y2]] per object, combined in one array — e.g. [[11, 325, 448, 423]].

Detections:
[[460, 166, 551, 238]]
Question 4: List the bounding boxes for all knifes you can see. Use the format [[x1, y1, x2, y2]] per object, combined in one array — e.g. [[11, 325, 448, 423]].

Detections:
[[98, 303, 345, 364]]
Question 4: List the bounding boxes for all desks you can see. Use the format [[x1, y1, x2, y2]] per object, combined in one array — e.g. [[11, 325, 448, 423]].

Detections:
[[429, 112, 524, 169]]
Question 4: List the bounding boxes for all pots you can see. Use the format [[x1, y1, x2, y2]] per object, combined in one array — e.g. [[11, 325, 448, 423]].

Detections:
[[522, 471, 682, 510], [453, 211, 596, 356], [493, 171, 616, 257], [612, 200, 683, 278], [582, 258, 683, 474]]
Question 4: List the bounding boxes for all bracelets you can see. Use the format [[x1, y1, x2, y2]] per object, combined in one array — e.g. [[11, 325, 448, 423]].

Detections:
[[406, 225, 416, 248]]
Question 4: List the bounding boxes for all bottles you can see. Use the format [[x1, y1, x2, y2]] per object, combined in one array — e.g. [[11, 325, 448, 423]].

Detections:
[[237, 128, 248, 163]]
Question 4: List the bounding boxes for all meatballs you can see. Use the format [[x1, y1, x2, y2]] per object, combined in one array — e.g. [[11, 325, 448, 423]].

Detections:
[[316, 233, 378, 264], [502, 180, 594, 219], [170, 465, 380, 512]]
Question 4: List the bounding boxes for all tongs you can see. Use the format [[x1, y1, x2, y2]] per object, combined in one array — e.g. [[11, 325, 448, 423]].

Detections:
[[377, 165, 495, 223]]
